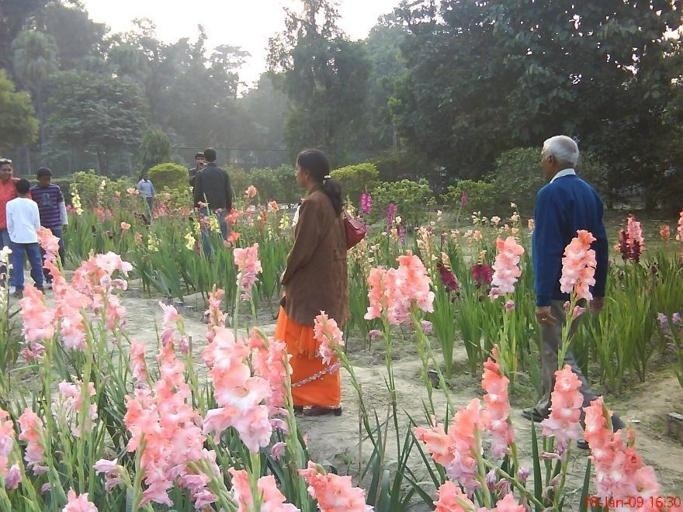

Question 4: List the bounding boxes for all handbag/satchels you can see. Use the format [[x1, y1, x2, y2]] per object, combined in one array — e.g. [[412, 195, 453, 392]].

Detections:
[[343, 207, 366, 251]]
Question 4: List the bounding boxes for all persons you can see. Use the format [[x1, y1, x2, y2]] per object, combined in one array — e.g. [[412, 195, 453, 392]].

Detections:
[[188, 152, 206, 191], [192, 146, 234, 267], [29, 166, 68, 291], [520, 133, 626, 449], [0, 157, 32, 289], [135, 169, 154, 225], [278, 147, 348, 420], [4, 177, 45, 298]]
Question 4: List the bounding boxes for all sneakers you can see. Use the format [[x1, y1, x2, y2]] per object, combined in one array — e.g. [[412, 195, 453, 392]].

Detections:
[[38, 287, 46, 295], [293, 402, 340, 415], [577, 439, 589, 449], [522, 405, 543, 423], [12, 290, 23, 298]]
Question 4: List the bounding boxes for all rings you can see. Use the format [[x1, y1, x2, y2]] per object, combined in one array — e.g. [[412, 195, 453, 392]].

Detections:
[[539, 315, 548, 322]]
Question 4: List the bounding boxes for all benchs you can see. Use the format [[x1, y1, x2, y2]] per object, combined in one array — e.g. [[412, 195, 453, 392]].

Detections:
[[134, 213, 151, 229]]
